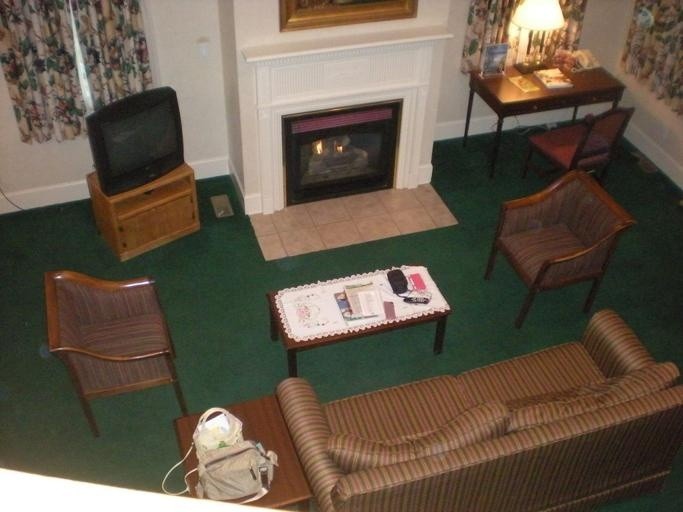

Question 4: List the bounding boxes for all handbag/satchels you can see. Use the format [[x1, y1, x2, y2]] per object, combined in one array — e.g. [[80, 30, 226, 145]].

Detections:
[[197, 441, 262, 501], [193, 407, 244, 458]]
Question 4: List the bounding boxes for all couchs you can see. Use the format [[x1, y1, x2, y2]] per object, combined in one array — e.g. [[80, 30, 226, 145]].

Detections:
[[275, 309, 683, 512]]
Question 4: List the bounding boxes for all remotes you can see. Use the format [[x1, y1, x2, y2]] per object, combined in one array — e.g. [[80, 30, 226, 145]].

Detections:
[[404, 297, 429, 303]]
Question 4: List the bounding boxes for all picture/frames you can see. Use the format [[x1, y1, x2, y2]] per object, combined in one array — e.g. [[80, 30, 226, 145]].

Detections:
[[278, 0, 418, 32]]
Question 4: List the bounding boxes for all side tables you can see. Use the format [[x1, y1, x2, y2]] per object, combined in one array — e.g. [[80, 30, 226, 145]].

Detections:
[[173, 394, 312, 512]]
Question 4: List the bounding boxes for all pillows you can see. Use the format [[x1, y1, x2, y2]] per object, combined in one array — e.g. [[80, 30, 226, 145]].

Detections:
[[505, 360, 680, 436], [327, 398, 512, 476]]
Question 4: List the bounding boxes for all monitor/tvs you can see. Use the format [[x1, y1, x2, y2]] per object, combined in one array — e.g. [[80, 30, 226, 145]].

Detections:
[[86, 86, 184, 197]]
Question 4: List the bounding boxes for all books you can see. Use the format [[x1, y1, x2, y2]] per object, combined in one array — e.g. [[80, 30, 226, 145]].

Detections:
[[508, 75, 539, 92], [533, 68, 574, 88]]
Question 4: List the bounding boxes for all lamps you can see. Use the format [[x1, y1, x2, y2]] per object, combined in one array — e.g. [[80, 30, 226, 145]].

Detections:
[[512, 0, 564, 73]]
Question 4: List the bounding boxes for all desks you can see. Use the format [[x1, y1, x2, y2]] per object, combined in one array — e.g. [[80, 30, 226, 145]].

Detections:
[[464, 63, 626, 176]]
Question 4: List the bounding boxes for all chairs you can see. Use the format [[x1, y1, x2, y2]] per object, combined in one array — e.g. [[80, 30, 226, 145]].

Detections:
[[483, 169, 638, 327], [44, 270, 188, 436], [522, 107, 635, 177]]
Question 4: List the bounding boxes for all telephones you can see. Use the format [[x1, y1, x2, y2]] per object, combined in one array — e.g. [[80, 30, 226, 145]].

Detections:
[[576, 48, 600, 70]]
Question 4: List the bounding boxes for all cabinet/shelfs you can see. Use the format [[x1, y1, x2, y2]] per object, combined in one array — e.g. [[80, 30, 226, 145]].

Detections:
[[86, 163, 201, 262]]
